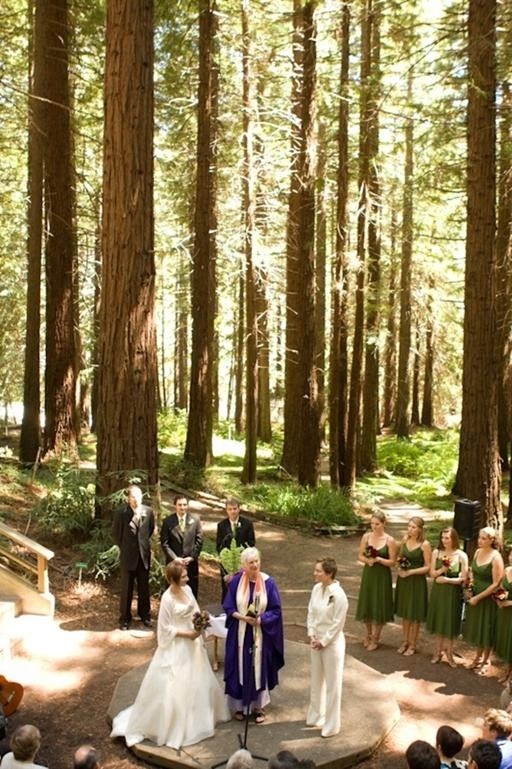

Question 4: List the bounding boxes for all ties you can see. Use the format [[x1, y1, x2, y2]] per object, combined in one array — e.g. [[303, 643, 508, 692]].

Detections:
[[179, 519, 183, 532], [232, 523, 237, 535]]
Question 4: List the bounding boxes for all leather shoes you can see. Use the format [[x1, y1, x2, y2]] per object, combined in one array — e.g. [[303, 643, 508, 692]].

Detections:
[[143, 619, 153, 628], [121, 621, 130, 630]]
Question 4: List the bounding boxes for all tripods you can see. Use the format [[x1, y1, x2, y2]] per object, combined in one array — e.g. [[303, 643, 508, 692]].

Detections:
[[210, 614, 268, 768]]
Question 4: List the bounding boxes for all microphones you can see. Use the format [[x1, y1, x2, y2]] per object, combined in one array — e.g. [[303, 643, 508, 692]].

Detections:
[[254, 592, 260, 613]]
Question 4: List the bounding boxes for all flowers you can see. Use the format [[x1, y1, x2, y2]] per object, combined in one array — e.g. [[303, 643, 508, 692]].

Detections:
[[494, 582, 510, 604], [395, 553, 415, 569], [191, 610, 212, 635], [436, 556, 458, 580], [363, 541, 381, 561], [462, 565, 476, 592]]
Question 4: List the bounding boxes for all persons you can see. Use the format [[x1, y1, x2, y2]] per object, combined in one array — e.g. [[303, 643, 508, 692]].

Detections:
[[110, 486, 155, 630], [223, 547, 285, 723], [2, 724, 50, 768], [73, 744, 101, 768], [225, 747, 316, 768], [430, 528, 468, 668], [464, 528, 503, 675], [357, 511, 397, 651], [496, 549, 512, 687], [396, 516, 431, 655], [217, 499, 255, 604], [161, 494, 203, 600], [406, 707, 511, 768], [110, 563, 232, 750], [304, 555, 349, 738]]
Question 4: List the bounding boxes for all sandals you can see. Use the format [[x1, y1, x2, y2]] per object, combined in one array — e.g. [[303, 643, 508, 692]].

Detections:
[[236, 710, 245, 721], [256, 708, 266, 723], [365, 634, 490, 675]]
